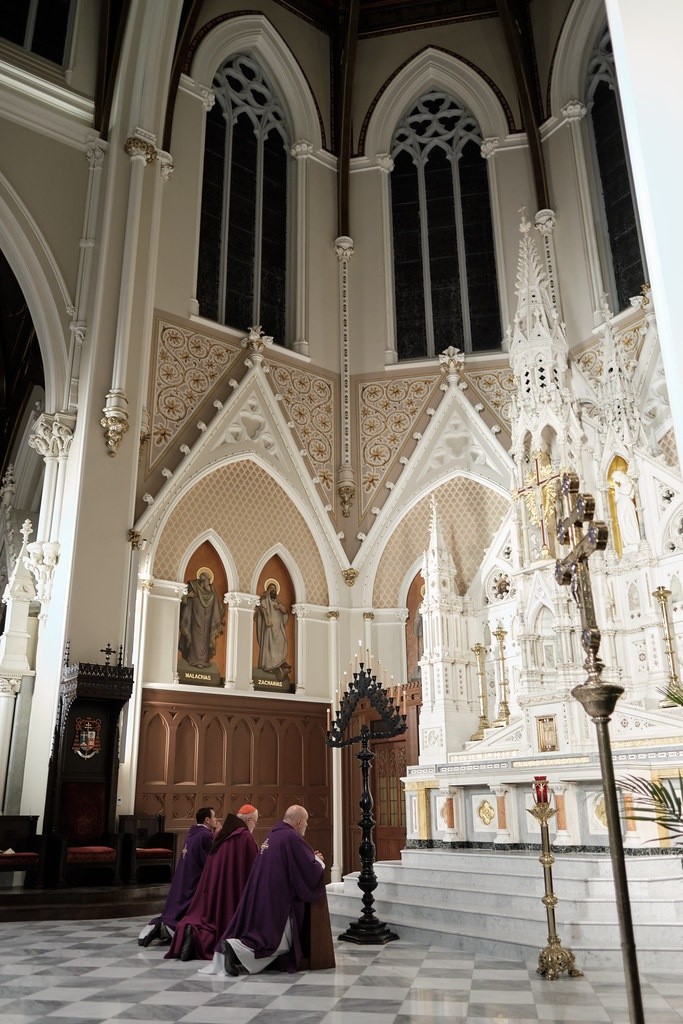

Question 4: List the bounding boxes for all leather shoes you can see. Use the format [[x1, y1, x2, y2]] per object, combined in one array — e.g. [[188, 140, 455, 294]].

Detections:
[[220, 941, 239, 975], [181, 926, 195, 963]]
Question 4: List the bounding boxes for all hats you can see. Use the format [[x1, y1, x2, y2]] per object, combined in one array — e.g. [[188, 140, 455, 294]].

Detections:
[[238, 804, 256, 814]]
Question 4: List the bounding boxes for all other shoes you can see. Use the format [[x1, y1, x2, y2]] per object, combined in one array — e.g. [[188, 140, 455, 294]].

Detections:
[[138, 917, 164, 946]]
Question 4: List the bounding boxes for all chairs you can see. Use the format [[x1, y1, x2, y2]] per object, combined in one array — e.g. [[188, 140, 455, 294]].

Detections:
[[35, 638, 134, 885], [118, 814, 178, 883], [0, 813, 41, 893]]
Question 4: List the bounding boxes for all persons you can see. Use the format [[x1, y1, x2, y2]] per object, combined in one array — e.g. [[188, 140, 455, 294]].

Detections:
[[255, 585, 293, 669], [181, 573, 226, 668], [165, 803, 258, 959], [137, 808, 221, 946], [215, 805, 325, 977]]
